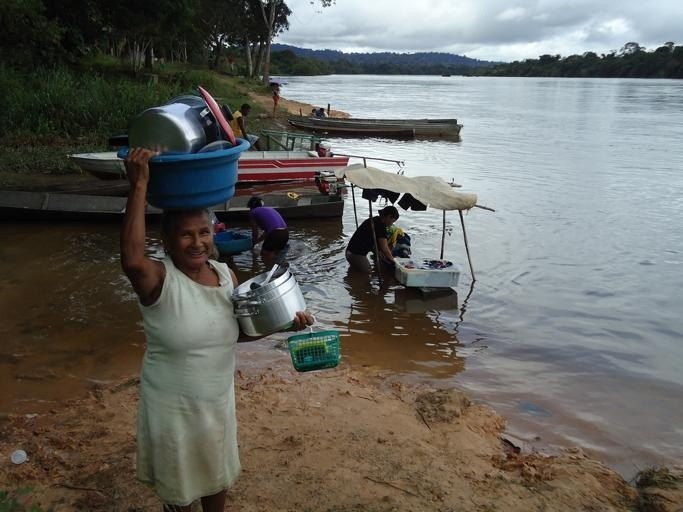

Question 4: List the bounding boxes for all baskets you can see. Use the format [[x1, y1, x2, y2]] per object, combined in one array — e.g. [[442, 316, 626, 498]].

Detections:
[[287, 330, 340, 372]]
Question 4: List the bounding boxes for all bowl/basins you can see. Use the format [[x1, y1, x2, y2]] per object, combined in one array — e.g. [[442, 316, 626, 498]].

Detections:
[[117, 138, 251, 211]]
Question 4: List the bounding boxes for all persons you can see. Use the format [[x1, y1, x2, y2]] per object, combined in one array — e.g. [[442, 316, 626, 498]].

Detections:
[[318, 108, 328, 118], [206, 55, 214, 70], [116, 146, 314, 511], [244, 197, 289, 258], [272, 92, 281, 114], [227, 103, 262, 152], [306, 109, 318, 118], [345, 205, 406, 274], [226, 54, 234, 73]]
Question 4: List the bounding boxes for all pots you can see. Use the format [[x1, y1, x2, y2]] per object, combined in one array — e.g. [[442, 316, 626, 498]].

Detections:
[[126, 103, 211, 155], [233, 266, 308, 338]]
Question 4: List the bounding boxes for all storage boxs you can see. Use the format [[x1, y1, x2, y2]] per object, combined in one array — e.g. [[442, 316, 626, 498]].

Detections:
[[394, 256, 461, 287]]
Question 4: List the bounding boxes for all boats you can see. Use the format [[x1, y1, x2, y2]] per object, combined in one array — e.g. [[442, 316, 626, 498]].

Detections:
[[0, 189, 345, 223], [308, 117, 464, 140], [65, 150, 351, 189]]
